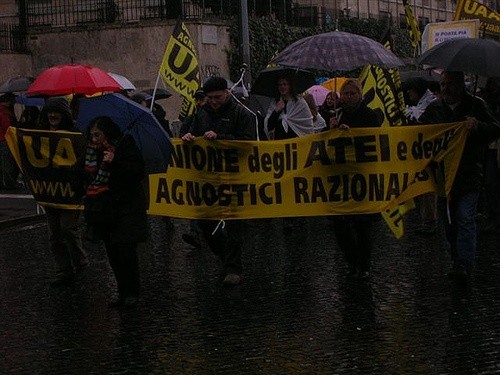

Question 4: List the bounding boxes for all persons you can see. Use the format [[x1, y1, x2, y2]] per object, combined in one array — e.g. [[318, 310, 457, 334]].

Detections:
[[0, 69, 499, 249], [413, 65, 499, 293], [181, 75, 257, 286], [27, 95, 93, 291], [82, 114, 145, 309], [319, 78, 381, 281]]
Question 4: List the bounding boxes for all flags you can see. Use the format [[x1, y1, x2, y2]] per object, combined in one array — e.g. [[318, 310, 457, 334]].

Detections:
[[452, 0, 499, 40], [352, 28, 410, 128], [402, 0, 422, 49], [158, 13, 204, 128]]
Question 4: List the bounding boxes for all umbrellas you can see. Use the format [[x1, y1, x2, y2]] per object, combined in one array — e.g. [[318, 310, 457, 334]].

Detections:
[[416, 37, 500, 122], [270, 27, 407, 124], [25, 62, 125, 130]]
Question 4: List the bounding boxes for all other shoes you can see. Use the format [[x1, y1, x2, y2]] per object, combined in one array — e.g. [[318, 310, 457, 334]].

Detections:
[[182, 232, 201, 248], [223, 274, 241, 285], [444, 265, 470, 280], [344, 267, 370, 279]]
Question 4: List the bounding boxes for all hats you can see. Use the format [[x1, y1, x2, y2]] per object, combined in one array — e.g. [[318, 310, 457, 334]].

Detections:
[[203, 75, 227, 95], [46, 96, 72, 118], [192, 88, 206, 97]]
